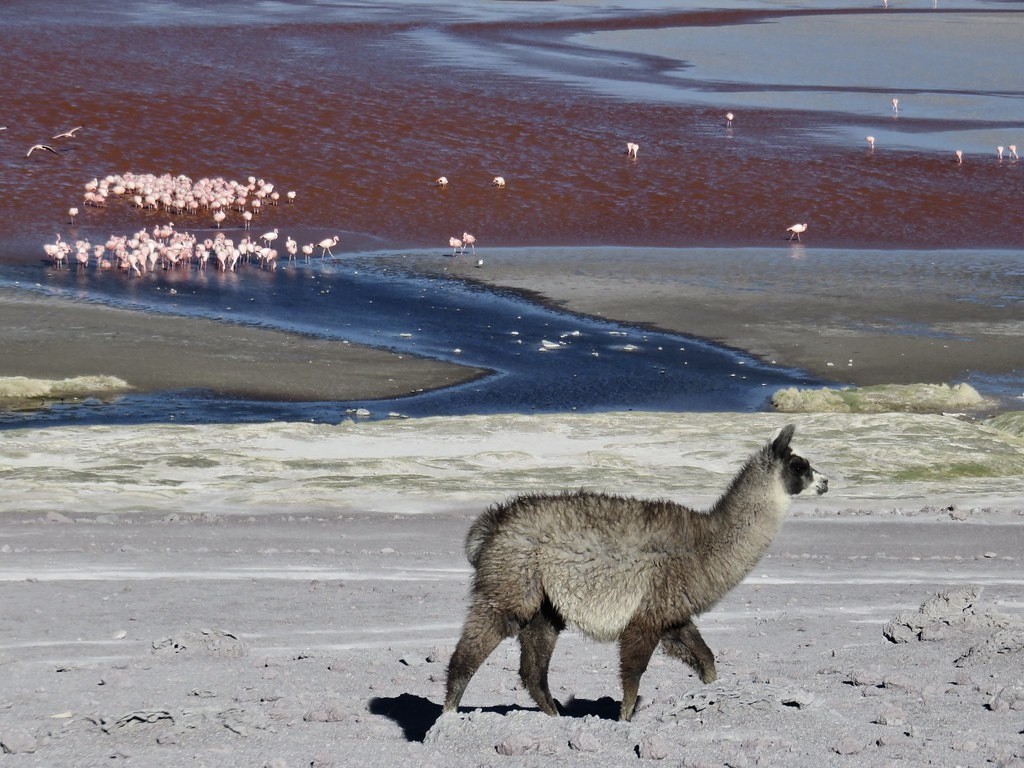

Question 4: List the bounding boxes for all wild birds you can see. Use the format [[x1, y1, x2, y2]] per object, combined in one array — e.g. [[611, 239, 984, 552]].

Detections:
[[24, 123, 82, 158], [42, 171, 340, 278], [725, 112, 734, 127], [626, 142, 639, 159], [786, 223, 808, 243], [954, 150, 963, 165], [865, 135, 875, 150], [449, 232, 477, 259], [892, 98, 899, 113], [997, 145, 1019, 161], [493, 176, 505, 188], [435, 176, 449, 189]]
